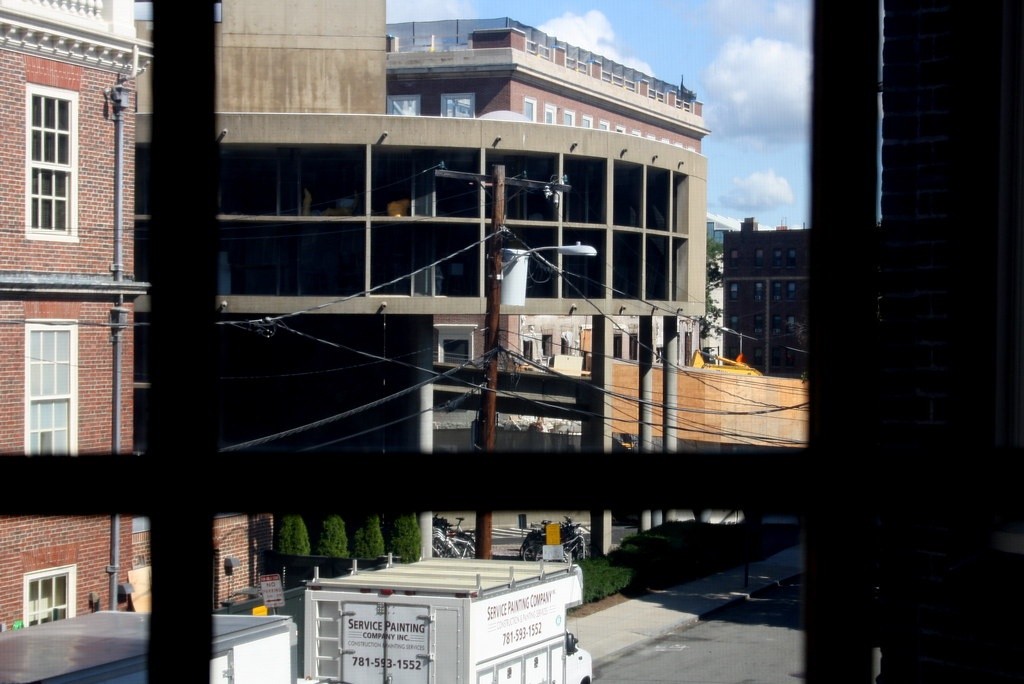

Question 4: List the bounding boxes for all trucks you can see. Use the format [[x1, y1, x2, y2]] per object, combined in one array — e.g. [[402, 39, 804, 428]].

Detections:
[[292, 551, 596, 684]]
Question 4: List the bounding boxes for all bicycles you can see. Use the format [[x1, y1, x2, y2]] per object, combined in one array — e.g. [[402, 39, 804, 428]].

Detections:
[[518, 515, 590, 561], [432, 514, 477, 560]]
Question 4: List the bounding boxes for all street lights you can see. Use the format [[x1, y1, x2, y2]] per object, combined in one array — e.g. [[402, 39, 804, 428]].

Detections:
[[476, 242, 598, 557]]
